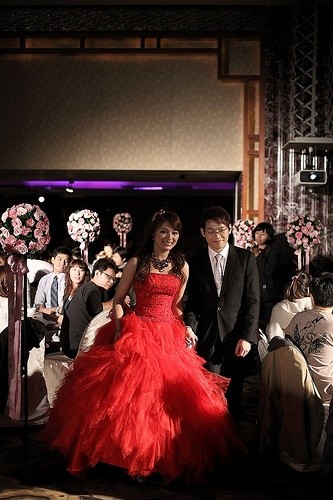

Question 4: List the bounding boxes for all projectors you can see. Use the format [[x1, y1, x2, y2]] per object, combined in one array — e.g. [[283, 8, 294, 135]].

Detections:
[[291, 170, 328, 187]]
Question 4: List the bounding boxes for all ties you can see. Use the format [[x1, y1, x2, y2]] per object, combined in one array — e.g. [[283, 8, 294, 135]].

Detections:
[[214, 254, 223, 297], [51, 276, 58, 307]]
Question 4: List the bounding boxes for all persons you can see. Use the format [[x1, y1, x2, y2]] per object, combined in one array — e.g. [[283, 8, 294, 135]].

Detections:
[[247, 223, 281, 340], [266, 270, 315, 346], [0, 245, 135, 362], [285, 272, 333, 428], [183, 206, 260, 430], [41, 211, 231, 486]]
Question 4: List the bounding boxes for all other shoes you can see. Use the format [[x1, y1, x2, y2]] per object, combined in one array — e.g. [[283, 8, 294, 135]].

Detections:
[[137, 476, 145, 482]]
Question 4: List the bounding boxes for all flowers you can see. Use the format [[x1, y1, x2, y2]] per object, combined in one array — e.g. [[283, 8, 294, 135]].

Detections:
[[0, 203, 51, 258], [285, 217, 324, 250], [66, 209, 101, 243], [232, 219, 256, 243], [113, 212, 133, 234]]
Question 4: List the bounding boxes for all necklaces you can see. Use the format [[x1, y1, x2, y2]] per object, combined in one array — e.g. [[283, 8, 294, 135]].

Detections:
[[150, 256, 170, 272]]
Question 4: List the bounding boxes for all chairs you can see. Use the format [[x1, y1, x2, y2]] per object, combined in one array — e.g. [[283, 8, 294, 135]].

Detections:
[[246, 328, 325, 474], [27, 304, 131, 424]]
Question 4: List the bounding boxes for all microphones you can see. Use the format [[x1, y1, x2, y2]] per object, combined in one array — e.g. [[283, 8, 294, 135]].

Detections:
[[251, 241, 258, 250]]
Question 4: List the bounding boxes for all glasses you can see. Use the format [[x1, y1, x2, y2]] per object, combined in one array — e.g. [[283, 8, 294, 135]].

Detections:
[[99, 270, 117, 282], [204, 227, 228, 234]]
